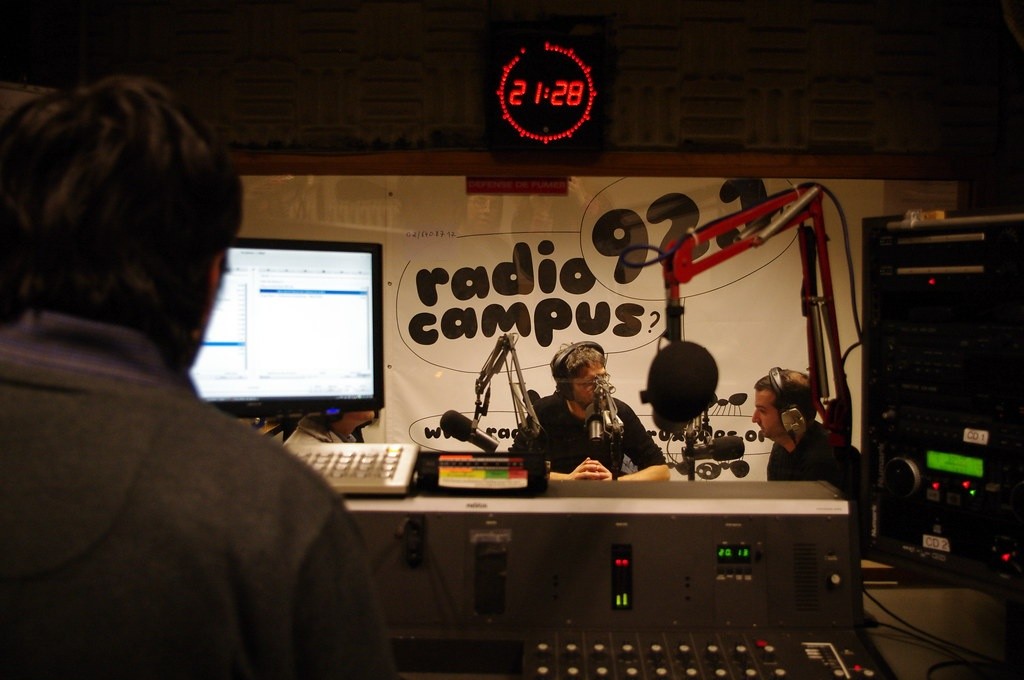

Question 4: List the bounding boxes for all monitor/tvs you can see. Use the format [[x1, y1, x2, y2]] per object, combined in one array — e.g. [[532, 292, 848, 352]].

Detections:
[[189, 239, 384, 416]]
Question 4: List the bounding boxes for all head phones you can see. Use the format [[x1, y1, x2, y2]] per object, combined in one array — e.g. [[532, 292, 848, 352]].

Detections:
[[552, 342, 604, 401], [770, 366, 808, 437]]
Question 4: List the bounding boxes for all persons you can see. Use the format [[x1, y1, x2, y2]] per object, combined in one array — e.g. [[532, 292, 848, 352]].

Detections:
[[513, 341, 670, 482], [283, 411, 375, 444], [752, 367, 872, 555], [0, 74, 396, 680]]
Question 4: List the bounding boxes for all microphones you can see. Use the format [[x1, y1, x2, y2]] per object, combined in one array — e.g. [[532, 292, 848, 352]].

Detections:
[[688, 436, 745, 461], [647, 342, 718, 430], [440, 410, 500, 452], [584, 404, 605, 443]]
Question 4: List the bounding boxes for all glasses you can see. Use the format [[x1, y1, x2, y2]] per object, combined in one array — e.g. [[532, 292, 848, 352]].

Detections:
[[567, 373, 611, 387]]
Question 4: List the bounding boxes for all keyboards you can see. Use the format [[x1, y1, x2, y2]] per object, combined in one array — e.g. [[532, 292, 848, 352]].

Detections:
[[286, 443, 421, 496]]
